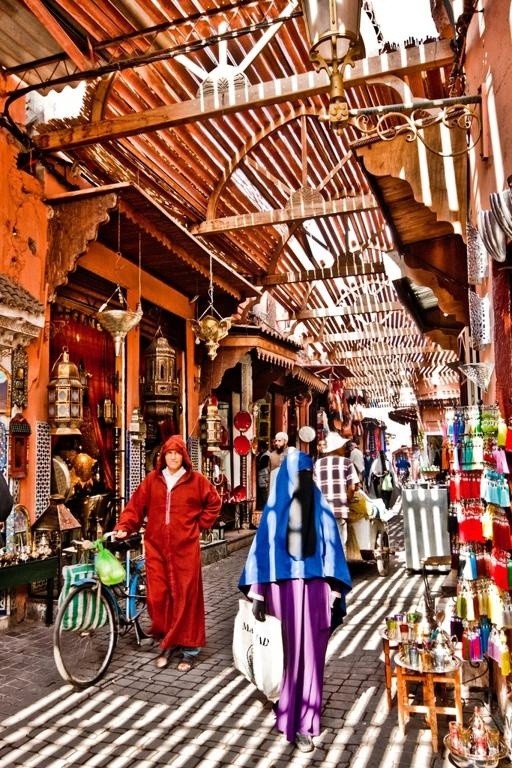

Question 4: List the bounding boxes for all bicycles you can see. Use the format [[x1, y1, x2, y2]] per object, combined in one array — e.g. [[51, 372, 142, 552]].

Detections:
[[53, 531, 157, 689]]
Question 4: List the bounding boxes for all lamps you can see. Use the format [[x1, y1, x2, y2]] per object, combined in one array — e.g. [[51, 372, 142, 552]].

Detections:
[[47, 344, 85, 436], [200, 396, 223, 451], [95, 203, 233, 361], [301, 0, 482, 156]]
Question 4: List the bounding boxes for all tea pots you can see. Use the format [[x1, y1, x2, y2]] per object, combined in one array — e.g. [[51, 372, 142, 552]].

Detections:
[[428, 628, 459, 659]]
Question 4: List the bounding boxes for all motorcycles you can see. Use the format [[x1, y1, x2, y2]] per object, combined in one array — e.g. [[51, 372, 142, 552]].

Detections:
[[395, 465, 410, 485]]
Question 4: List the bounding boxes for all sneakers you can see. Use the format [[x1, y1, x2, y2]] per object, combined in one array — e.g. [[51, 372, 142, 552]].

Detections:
[[295, 731, 315, 752]]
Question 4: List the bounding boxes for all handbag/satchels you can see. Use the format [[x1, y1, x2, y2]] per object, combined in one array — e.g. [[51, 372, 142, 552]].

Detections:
[[58, 562, 109, 633], [382, 475, 393, 492], [230, 599, 285, 706]]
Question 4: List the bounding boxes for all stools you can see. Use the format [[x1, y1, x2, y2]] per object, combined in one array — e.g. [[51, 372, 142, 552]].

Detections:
[[419, 555, 452, 604], [381, 621, 464, 755]]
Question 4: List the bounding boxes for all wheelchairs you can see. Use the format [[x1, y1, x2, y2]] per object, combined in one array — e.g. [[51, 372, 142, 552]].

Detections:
[[347, 490, 395, 577]]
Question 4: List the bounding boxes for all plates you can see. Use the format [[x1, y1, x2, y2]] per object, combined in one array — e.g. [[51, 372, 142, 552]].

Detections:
[[393, 649, 462, 674]]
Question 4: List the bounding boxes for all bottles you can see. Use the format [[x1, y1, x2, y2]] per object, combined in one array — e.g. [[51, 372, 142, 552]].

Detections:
[[385, 613, 417, 641]]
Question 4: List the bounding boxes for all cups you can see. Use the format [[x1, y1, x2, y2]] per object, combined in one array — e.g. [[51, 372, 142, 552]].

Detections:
[[401, 643, 452, 670]]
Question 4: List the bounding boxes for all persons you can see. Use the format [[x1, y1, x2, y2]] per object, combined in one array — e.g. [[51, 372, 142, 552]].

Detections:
[[236, 450, 351, 756], [311, 439, 327, 458], [113, 433, 222, 673], [341, 433, 412, 510], [269, 430, 295, 471], [259, 439, 269, 509], [314, 432, 360, 558]]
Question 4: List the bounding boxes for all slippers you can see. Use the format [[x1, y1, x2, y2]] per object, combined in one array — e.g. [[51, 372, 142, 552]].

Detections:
[[157, 645, 181, 668], [177, 656, 195, 672]]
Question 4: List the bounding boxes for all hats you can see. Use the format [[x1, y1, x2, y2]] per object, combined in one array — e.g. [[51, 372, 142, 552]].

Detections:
[[323, 431, 350, 454]]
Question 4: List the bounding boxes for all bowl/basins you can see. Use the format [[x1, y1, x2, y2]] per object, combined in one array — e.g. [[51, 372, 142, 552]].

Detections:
[[442, 732, 510, 768]]
